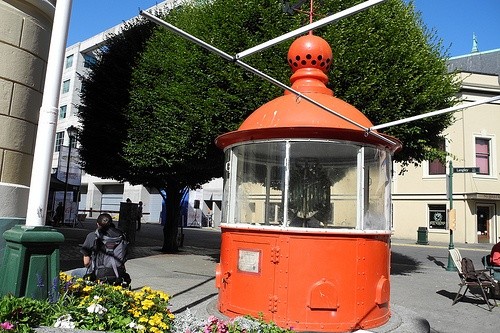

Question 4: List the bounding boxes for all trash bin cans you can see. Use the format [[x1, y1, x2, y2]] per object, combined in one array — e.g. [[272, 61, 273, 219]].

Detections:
[[416, 227, 429, 246]]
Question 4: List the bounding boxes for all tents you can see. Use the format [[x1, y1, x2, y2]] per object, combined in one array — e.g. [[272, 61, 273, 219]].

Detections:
[[46, 176, 81, 228]]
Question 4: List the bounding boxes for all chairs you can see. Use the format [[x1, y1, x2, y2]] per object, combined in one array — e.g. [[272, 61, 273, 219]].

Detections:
[[448, 249, 500, 311], [481, 255, 500, 283], [72, 214, 86, 228]]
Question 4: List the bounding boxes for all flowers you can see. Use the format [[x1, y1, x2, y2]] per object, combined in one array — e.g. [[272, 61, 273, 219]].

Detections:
[[0, 271, 297, 333]]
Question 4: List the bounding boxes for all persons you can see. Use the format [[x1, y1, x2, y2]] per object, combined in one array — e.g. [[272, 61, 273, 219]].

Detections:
[[489, 237, 500, 272], [126, 198, 143, 231], [80, 213, 130, 291], [53, 202, 63, 226]]
